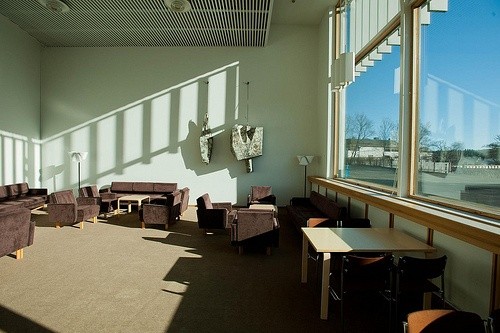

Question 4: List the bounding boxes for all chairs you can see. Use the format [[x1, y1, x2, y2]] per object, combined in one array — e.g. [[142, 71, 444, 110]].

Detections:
[[307, 218, 500, 333]]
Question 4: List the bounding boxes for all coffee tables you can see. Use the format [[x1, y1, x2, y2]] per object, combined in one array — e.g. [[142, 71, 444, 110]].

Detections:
[[117, 195, 150, 213]]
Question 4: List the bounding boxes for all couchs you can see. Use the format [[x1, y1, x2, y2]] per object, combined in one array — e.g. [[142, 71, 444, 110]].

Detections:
[[231, 211, 280, 256], [47, 189, 100, 230], [195, 193, 232, 234], [0, 208, 35, 260], [79, 185, 117, 220], [140, 187, 190, 230], [247, 185, 277, 207], [286, 192, 347, 238], [0, 183, 48, 210], [99, 182, 177, 209]]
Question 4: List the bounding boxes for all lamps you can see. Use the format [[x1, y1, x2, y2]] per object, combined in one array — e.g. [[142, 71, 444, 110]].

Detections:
[[297, 156, 314, 196], [69, 151, 88, 189]]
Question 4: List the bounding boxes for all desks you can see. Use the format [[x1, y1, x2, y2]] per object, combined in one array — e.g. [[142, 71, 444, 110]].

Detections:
[[248, 204, 276, 211], [301, 227, 436, 319]]
